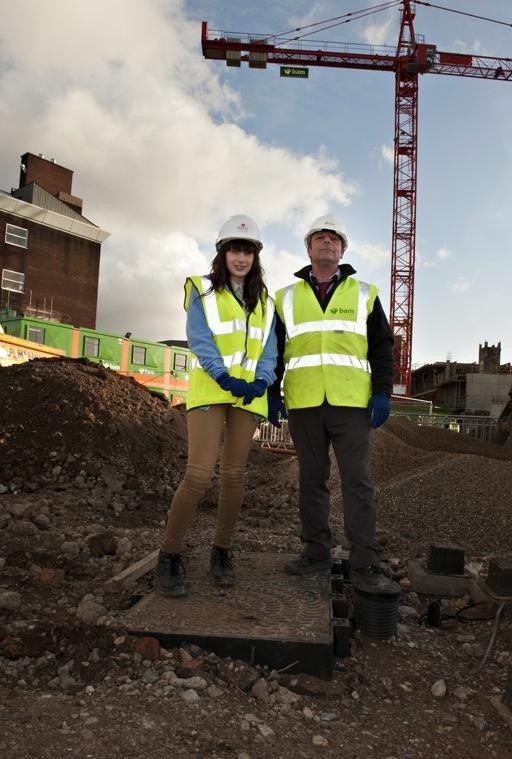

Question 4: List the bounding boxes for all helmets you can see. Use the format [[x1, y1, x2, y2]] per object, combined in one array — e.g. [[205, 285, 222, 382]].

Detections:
[[302, 214, 350, 255], [214, 214, 265, 254]]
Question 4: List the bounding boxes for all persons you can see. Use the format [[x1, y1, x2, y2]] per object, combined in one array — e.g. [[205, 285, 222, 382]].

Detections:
[[457, 416, 462, 432], [444, 416, 449, 431], [267, 215, 398, 594], [152, 212, 278, 600], [497, 385, 512, 450]]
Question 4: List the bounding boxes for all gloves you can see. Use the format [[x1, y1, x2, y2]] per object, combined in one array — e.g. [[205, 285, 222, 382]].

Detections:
[[267, 398, 289, 429], [215, 372, 248, 397], [242, 378, 268, 406], [364, 390, 392, 431]]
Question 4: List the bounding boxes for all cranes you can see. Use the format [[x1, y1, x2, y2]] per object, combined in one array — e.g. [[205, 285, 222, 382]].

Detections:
[[200, 2, 510, 401]]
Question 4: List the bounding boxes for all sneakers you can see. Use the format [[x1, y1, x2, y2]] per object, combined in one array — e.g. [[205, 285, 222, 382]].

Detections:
[[349, 561, 386, 592], [283, 541, 334, 578]]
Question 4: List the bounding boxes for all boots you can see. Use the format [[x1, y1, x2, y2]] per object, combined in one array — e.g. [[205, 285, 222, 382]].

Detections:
[[207, 541, 238, 589], [153, 546, 188, 598]]
